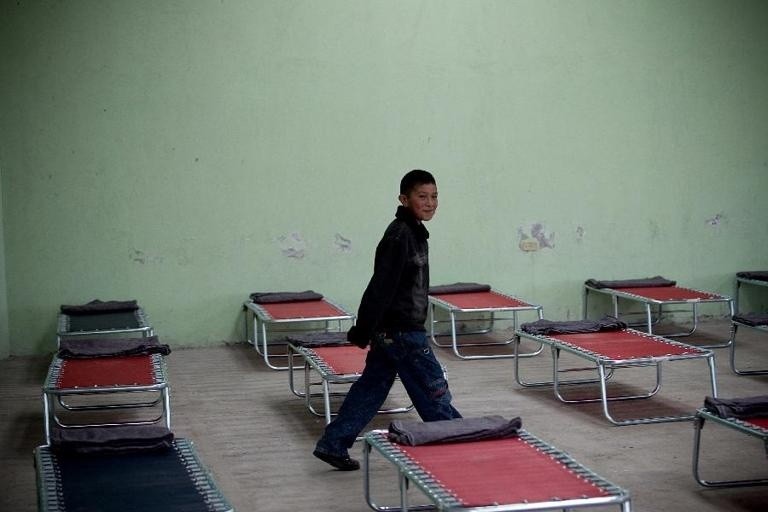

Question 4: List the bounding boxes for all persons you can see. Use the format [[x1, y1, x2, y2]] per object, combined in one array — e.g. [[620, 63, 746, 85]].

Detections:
[[309, 166, 463, 469]]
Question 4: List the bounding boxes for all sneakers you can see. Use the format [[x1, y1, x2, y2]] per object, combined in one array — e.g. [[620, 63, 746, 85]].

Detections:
[[313, 450, 360, 470]]
